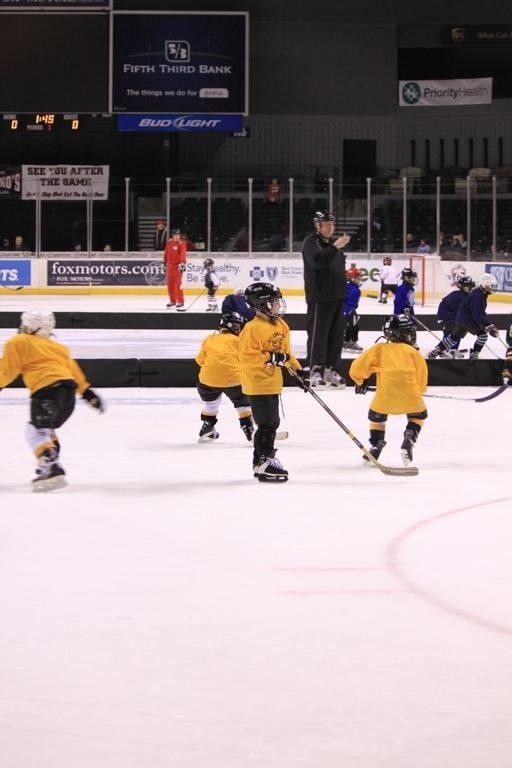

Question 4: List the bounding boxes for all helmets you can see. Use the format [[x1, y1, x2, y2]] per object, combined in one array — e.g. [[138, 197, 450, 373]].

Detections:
[[218, 294, 256, 335], [313, 210, 335, 223], [381, 313, 417, 345], [170, 227, 181, 234], [17, 308, 57, 338], [346, 267, 498, 296], [204, 259, 214, 267], [244, 281, 286, 322]]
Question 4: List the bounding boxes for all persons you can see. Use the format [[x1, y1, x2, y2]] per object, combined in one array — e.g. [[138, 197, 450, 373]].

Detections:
[[349, 314, 429, 468], [399, 232, 416, 253], [222, 276, 256, 321], [377, 258, 399, 303], [302, 213, 350, 390], [393, 268, 420, 351], [180, 230, 194, 251], [425, 273, 499, 359], [434, 231, 450, 253], [157, 219, 169, 251], [14, 235, 33, 251], [437, 276, 476, 359], [0, 307, 103, 482], [416, 238, 433, 253], [342, 267, 363, 353], [192, 312, 257, 442], [72, 244, 82, 252], [204, 259, 220, 311], [502, 319, 511, 388], [268, 177, 284, 205], [450, 233, 467, 255], [104, 245, 112, 251], [163, 228, 187, 308], [238, 283, 310, 478]]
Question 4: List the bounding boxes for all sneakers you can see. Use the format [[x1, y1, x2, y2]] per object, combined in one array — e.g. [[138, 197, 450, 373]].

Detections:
[[342, 339, 363, 351], [324, 368, 346, 385], [176, 303, 184, 307], [166, 302, 176, 306], [310, 370, 326, 386]]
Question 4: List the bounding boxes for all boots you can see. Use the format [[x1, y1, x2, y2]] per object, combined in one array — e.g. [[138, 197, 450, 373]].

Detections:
[[258, 461, 289, 476], [364, 439, 386, 462], [32, 455, 66, 483], [401, 430, 413, 461], [201, 417, 219, 440], [239, 421, 256, 440]]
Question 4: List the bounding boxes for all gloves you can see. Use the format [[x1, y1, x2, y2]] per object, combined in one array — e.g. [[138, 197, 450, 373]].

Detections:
[[268, 351, 290, 367], [484, 322, 499, 338], [296, 369, 310, 392], [82, 390, 104, 412], [354, 381, 368, 394], [178, 264, 186, 273]]
